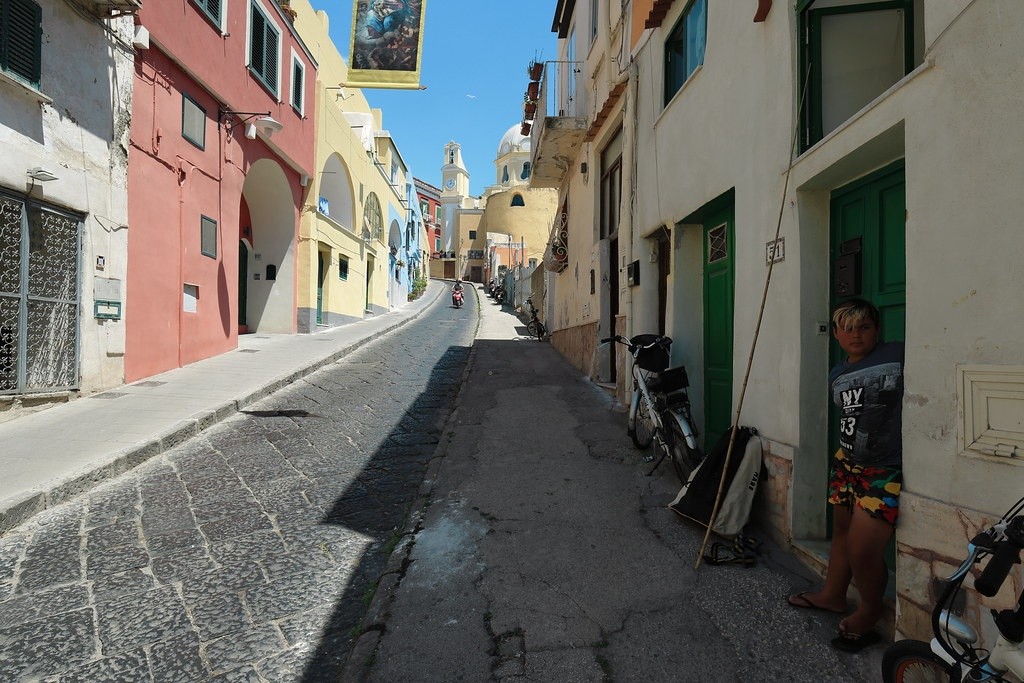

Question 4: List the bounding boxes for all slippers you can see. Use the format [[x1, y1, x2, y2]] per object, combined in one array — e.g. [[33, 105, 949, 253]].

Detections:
[[788, 591, 850, 614], [830, 630, 882, 651]]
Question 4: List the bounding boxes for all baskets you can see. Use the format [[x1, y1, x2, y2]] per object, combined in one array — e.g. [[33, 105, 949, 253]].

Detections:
[[630, 334, 673, 371]]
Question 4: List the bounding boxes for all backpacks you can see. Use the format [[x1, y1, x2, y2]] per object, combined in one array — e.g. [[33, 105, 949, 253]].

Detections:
[[668, 425, 764, 540]]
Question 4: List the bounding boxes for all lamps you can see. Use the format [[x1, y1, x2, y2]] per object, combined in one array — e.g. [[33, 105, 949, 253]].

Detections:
[[217, 105, 284, 139], [26, 168, 60, 182]]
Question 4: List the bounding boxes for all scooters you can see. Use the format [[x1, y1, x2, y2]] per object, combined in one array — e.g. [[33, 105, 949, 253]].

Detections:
[[880, 498, 1024, 683], [451, 288, 464, 308], [489, 279, 505, 304]]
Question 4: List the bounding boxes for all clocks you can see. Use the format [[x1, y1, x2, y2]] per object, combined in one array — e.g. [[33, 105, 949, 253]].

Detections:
[[445, 178, 456, 190]]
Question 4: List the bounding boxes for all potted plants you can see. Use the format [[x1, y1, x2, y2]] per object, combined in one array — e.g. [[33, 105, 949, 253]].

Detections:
[[521, 46, 546, 137], [390, 242, 427, 303]]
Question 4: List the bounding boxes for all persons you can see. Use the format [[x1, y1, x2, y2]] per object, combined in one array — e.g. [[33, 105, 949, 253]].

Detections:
[[786, 298, 905, 654], [451, 277, 464, 305]]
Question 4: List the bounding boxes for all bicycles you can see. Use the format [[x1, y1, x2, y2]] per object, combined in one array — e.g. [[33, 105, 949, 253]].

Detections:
[[596, 333, 702, 479], [523, 292, 545, 342]]
